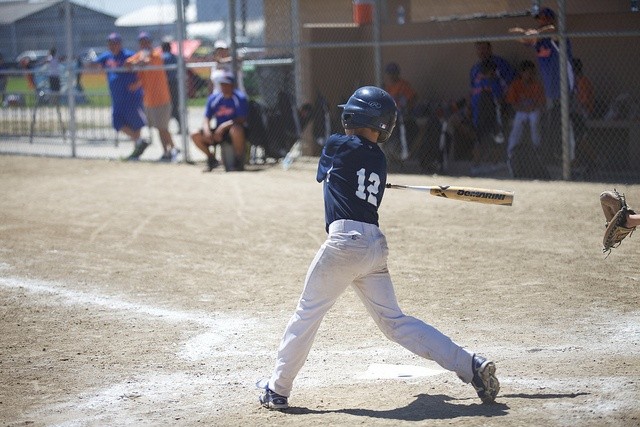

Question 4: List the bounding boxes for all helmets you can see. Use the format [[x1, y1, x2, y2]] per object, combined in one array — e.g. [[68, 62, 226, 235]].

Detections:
[[337, 86, 398, 143]]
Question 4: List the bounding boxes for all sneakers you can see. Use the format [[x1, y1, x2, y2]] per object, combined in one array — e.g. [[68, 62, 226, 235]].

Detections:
[[125, 155, 138, 160], [471, 356, 500, 405], [235, 153, 244, 171], [158, 155, 172, 161], [256, 380, 288, 410], [203, 159, 219, 172], [135, 140, 148, 158], [169, 148, 180, 158]]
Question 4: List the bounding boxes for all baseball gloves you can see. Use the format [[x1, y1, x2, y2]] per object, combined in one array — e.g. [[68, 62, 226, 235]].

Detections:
[[601, 191, 637, 259]]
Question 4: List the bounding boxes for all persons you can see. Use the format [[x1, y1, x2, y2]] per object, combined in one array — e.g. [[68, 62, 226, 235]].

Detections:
[[207, 39, 234, 95], [572, 57, 594, 115], [506, 61, 546, 181], [190, 71, 249, 171], [78, 33, 151, 159], [122, 31, 182, 163], [247, 93, 346, 157], [413, 67, 505, 173], [507, 7, 585, 176], [258, 85, 500, 411], [620, 212, 639, 229], [384, 62, 416, 160], [470, 37, 511, 155], [161, 36, 190, 136]]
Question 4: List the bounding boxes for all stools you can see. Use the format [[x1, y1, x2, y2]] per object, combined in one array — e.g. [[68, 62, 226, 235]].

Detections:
[[213, 136, 247, 171]]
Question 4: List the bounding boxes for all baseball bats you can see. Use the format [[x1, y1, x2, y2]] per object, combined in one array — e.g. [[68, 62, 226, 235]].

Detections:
[[385, 183, 514, 206]]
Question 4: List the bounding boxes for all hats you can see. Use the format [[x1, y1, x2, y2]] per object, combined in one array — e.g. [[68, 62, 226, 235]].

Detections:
[[215, 41, 227, 50], [385, 64, 398, 73], [138, 32, 154, 43], [107, 33, 121, 42], [534, 8, 555, 20], [219, 73, 234, 84]]
